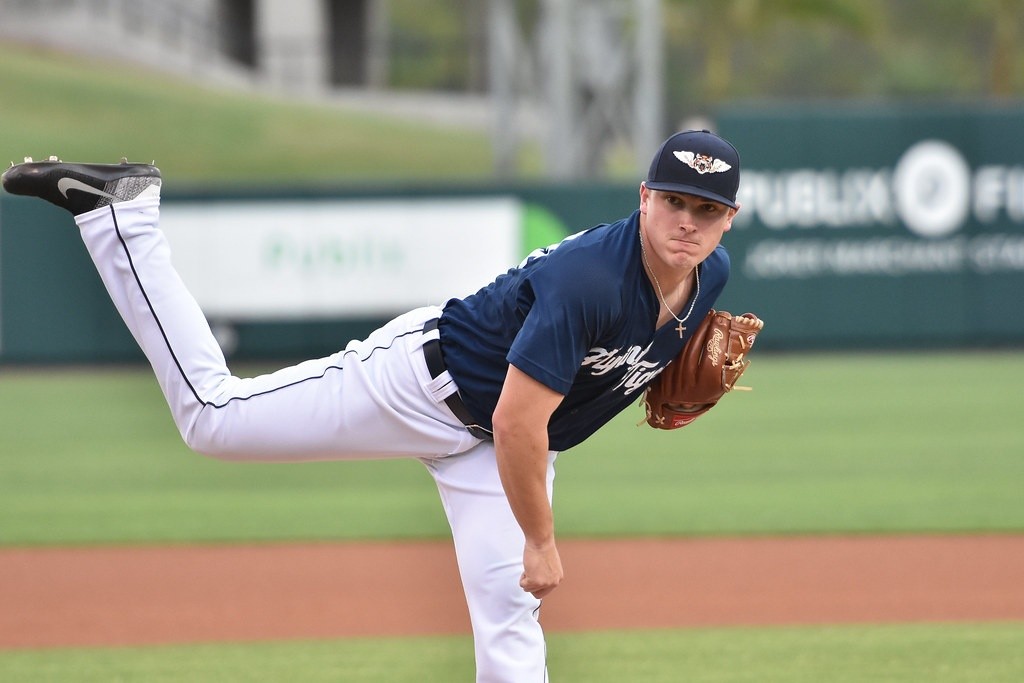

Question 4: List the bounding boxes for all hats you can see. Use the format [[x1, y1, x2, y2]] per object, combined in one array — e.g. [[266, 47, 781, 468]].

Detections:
[[646, 129, 740, 210]]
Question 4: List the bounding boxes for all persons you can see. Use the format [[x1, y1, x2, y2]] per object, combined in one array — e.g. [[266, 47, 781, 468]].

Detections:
[[3, 128, 766, 683]]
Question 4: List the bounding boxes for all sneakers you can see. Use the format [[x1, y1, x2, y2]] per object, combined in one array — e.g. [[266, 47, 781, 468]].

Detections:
[[1, 156, 163, 216]]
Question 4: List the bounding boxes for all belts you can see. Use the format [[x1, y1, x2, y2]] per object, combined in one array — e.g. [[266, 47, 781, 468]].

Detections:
[[422, 318, 494, 441]]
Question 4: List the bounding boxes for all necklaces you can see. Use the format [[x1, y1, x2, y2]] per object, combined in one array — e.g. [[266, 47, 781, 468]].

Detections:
[[637, 226, 701, 339]]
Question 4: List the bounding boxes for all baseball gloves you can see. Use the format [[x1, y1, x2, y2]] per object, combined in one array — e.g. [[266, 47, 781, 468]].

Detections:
[[637, 307, 765, 431]]
